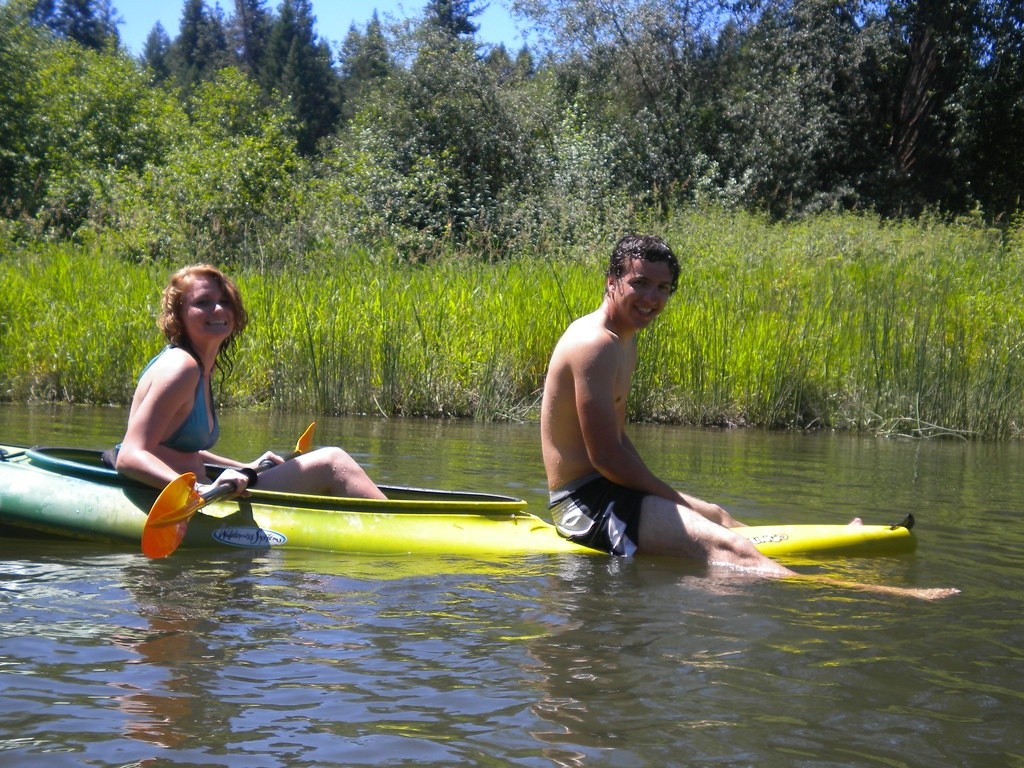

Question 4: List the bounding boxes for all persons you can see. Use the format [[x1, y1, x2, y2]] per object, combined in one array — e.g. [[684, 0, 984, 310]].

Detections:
[[114, 264, 387, 500], [541, 234, 961, 601]]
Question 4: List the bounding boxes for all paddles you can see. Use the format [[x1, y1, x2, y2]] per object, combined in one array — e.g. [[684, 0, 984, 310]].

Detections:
[[142, 420, 317, 562]]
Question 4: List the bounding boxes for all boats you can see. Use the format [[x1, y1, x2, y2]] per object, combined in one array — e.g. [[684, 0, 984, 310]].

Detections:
[[262, 549, 525, 576], [0, 443, 922, 555]]
